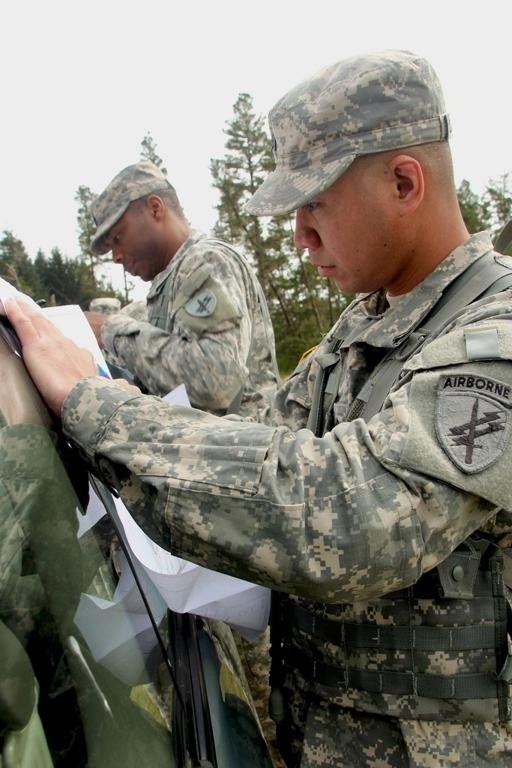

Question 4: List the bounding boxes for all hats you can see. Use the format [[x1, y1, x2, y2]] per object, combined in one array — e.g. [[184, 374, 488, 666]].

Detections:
[[89, 157, 171, 254], [89, 297, 122, 315], [244, 48, 451, 217]]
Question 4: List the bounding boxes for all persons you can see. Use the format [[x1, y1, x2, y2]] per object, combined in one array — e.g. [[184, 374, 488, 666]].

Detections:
[[0, 332, 180, 768], [79, 155, 292, 758], [2, 46, 512, 767]]
